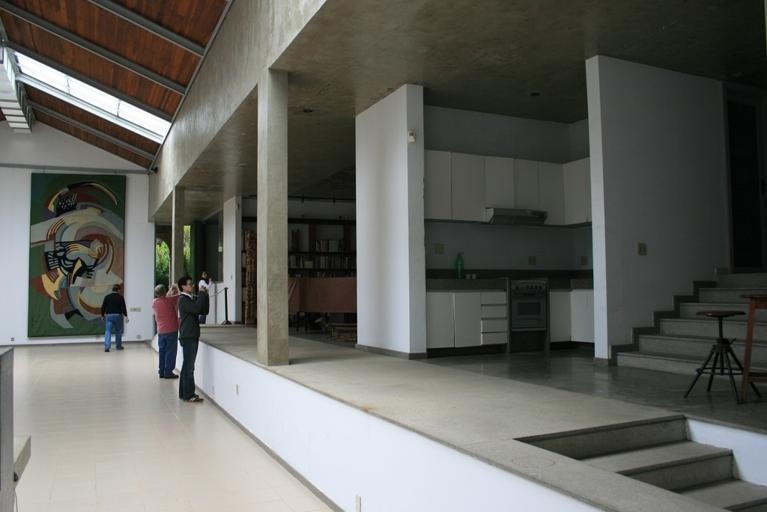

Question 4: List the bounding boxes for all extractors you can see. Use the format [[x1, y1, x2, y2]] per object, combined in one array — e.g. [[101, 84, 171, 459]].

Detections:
[[485, 208, 547, 225]]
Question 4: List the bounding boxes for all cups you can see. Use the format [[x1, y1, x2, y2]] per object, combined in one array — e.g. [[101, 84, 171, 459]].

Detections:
[[466, 273, 479, 280]]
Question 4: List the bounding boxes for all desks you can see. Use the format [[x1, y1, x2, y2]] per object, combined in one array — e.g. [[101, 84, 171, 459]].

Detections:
[[287, 277, 357, 334], [738, 292, 766, 405]]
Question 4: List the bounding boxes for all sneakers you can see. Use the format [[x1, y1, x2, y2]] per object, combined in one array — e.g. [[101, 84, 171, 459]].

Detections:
[[179, 394, 203, 403], [116, 345, 125, 350], [105, 349, 110, 352], [159, 372, 179, 379]]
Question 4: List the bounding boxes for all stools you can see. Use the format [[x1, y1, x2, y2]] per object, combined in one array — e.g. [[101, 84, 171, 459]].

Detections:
[[682, 309, 761, 405]]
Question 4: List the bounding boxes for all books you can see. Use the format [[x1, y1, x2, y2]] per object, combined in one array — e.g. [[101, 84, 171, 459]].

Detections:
[[290, 239, 356, 277]]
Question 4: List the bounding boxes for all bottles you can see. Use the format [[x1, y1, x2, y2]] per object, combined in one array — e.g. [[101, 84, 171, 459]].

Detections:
[[455, 254, 465, 280]]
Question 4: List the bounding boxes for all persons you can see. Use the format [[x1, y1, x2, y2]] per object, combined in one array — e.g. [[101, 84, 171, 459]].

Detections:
[[152, 284, 179, 378], [198, 271, 212, 324], [101, 284, 129, 352], [177, 276, 209, 403]]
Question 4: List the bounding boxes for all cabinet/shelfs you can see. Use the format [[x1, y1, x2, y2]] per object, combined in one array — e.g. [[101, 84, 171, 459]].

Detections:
[[560, 157, 592, 228], [478, 289, 508, 354], [484, 155, 539, 212], [549, 289, 570, 348], [568, 288, 594, 348], [424, 289, 481, 356], [423, 149, 485, 224], [536, 162, 564, 228], [241, 217, 357, 331]]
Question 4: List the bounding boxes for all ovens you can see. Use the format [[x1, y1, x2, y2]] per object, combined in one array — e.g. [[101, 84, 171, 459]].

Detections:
[[511, 296, 547, 330]]
